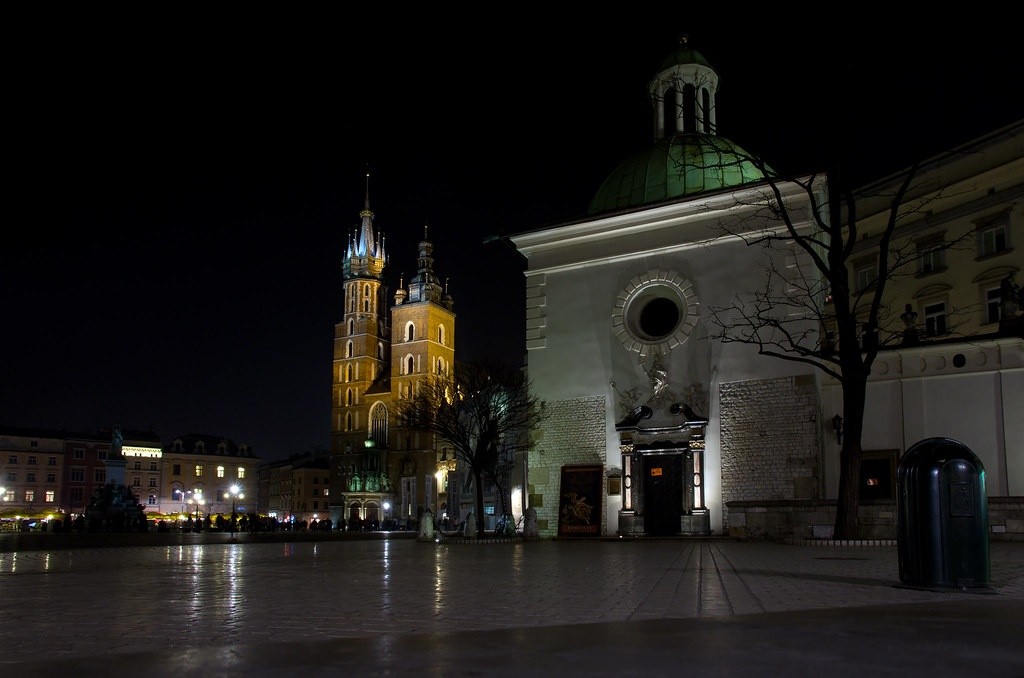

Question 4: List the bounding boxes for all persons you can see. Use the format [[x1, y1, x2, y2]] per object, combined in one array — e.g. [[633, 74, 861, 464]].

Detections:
[[188, 511, 420, 533], [436, 516, 449, 531], [620, 354, 706, 417]]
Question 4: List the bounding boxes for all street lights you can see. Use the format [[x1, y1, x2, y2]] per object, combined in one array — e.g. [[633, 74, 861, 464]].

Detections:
[[224, 484, 246, 527], [189, 493, 205, 522]]
